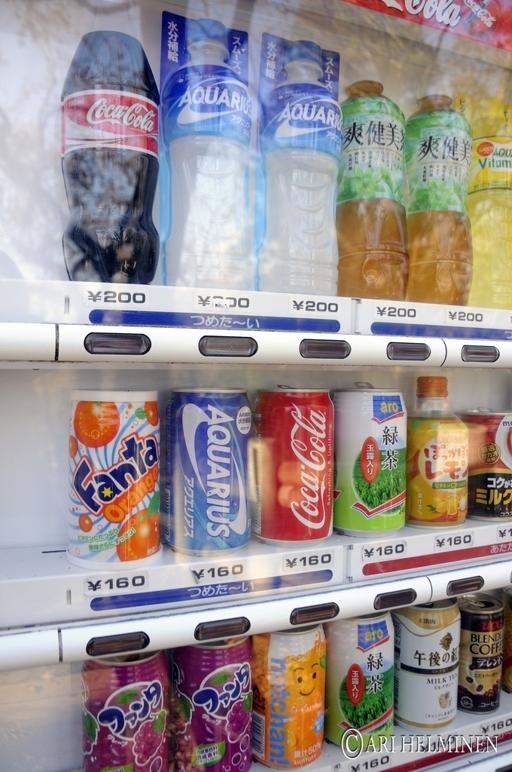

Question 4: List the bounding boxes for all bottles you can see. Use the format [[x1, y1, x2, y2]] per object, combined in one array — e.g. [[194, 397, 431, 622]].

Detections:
[[405, 373, 468, 522], [60, 1, 512, 308]]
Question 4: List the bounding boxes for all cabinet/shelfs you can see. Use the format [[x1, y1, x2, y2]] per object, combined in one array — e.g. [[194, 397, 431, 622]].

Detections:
[[0, 272, 512, 772]]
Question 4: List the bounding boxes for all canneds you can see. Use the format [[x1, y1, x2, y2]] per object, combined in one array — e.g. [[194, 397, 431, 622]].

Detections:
[[502, 586, 511, 694], [79, 650, 170, 772], [457, 593, 505, 714], [325, 611, 394, 752], [163, 386, 251, 556], [64, 387, 162, 570], [334, 389, 407, 537], [391, 597, 462, 732], [251, 624, 327, 769], [461, 408, 512, 522], [171, 636, 253, 772], [253, 385, 335, 546]]
